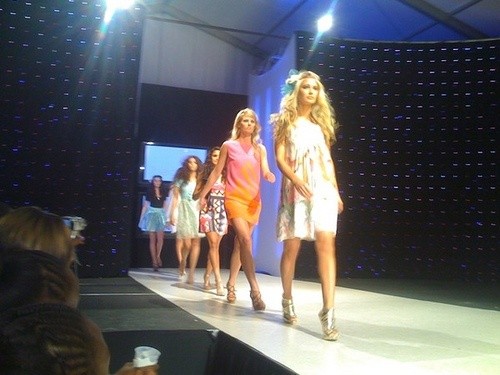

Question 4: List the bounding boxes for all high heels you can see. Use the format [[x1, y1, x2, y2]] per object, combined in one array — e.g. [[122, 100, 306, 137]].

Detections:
[[318, 307, 338, 341], [215, 279, 225, 296], [226, 281, 237, 302], [281, 293, 298, 323], [152, 260, 163, 272], [203, 274, 211, 289], [250, 290, 265, 311]]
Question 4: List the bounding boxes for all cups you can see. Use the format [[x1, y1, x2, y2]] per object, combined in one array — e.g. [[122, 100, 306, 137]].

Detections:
[[132, 346, 162, 369]]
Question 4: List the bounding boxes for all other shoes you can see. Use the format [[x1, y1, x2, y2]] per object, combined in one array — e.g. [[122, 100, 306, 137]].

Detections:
[[176, 267, 194, 284]]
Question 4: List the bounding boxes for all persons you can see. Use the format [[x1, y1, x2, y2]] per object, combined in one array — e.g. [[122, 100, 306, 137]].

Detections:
[[192, 146, 229, 295], [0, 206, 159, 375], [162, 155, 204, 285], [198, 108, 276, 311], [138, 175, 169, 272], [268, 69, 343, 340]]
